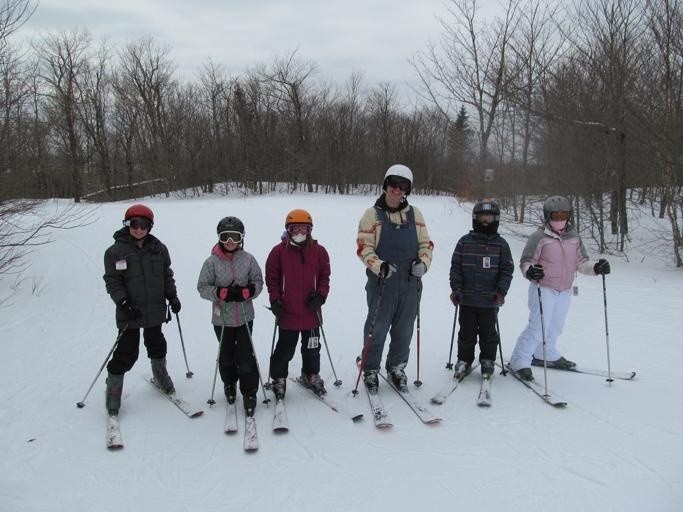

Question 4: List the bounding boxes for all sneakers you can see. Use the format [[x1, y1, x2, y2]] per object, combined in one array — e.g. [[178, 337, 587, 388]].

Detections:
[[454, 359, 471, 380], [224, 382, 237, 404], [480, 357, 494, 377], [517, 367, 534, 381], [271, 377, 286, 400], [244, 390, 257, 410], [363, 370, 379, 391], [531, 354, 576, 369], [302, 372, 326, 394], [388, 368, 408, 388]]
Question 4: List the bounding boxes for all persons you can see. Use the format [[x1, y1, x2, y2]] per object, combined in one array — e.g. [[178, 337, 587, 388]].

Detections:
[[355, 163, 434, 394], [508, 195, 611, 382], [195, 216, 264, 419], [265, 208, 332, 401], [102, 204, 181, 419], [448, 198, 515, 379]]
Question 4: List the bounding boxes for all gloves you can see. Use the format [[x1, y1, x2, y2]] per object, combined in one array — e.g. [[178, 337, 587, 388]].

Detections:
[[492, 290, 505, 307], [169, 297, 182, 314], [594, 258, 611, 275], [217, 279, 255, 303], [121, 302, 141, 320], [527, 264, 545, 282], [306, 292, 325, 310], [379, 261, 398, 279], [409, 259, 425, 278], [271, 299, 286, 316], [450, 290, 464, 305]]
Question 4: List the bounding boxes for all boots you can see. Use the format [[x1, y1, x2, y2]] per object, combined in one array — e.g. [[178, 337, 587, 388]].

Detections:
[[150, 357, 174, 393], [105, 370, 124, 415]]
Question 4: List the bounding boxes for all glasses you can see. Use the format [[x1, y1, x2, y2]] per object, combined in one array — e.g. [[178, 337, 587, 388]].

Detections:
[[550, 211, 570, 222], [126, 220, 153, 230], [218, 230, 242, 244], [286, 223, 312, 235], [386, 177, 410, 192]]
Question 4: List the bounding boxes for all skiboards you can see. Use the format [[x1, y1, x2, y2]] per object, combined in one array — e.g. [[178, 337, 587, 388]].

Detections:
[[357, 355, 442, 428], [496, 360, 636, 407], [432, 360, 492, 407], [273, 374, 364, 432], [108, 374, 203, 449], [224, 401, 258, 448]]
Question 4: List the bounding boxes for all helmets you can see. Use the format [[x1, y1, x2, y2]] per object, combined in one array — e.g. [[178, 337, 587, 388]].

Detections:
[[124, 204, 154, 222], [382, 163, 414, 197], [543, 196, 573, 223], [285, 208, 313, 229], [472, 201, 500, 228], [216, 216, 245, 234]]
